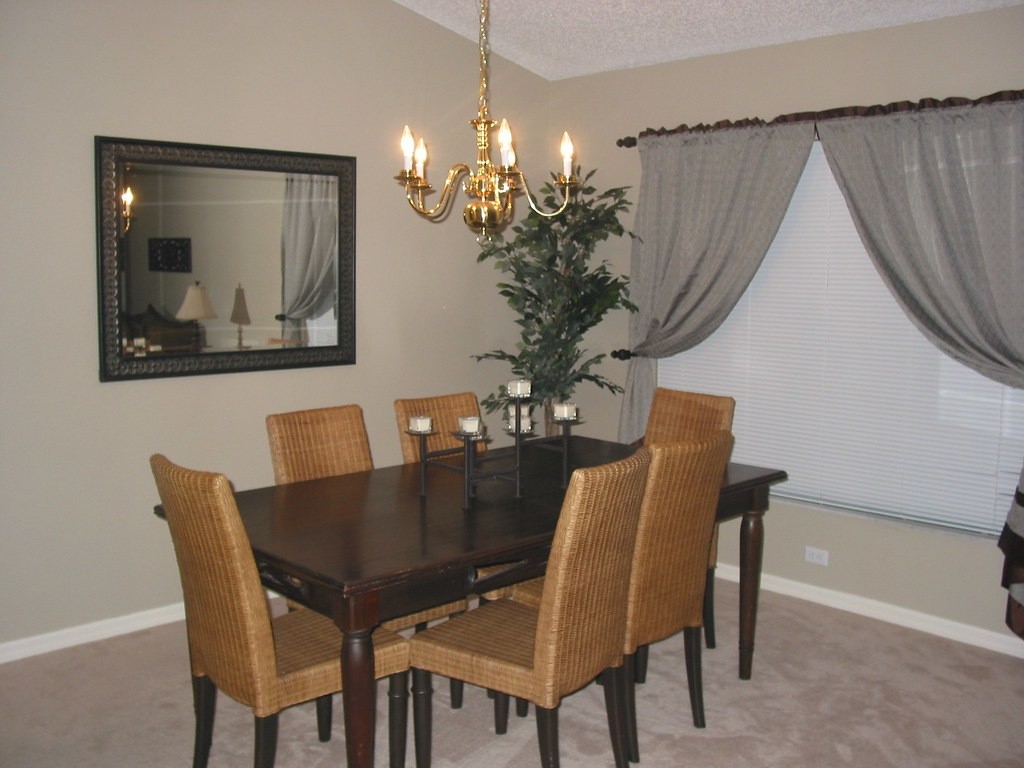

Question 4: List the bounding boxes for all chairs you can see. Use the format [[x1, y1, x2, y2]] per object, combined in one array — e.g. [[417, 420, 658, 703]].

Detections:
[[149, 454, 410, 768], [641, 386, 735, 649], [265, 403, 467, 706], [394, 393, 545, 699], [513, 430, 735, 729], [408, 446, 653, 768]]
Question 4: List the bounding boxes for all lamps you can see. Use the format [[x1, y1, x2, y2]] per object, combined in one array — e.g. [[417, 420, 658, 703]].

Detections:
[[394, 0, 573, 247]]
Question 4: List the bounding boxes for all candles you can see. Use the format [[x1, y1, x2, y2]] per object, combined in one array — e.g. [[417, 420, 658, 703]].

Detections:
[[458, 416, 480, 435], [554, 402, 577, 420], [507, 380, 531, 398], [508, 404, 530, 417], [409, 416, 432, 434], [509, 415, 532, 432]]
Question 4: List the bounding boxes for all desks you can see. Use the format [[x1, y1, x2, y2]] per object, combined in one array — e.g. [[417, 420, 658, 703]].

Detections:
[[154, 435, 788, 768]]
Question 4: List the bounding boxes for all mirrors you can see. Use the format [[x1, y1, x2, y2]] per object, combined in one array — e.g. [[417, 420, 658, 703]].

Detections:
[[94, 136, 357, 382]]
